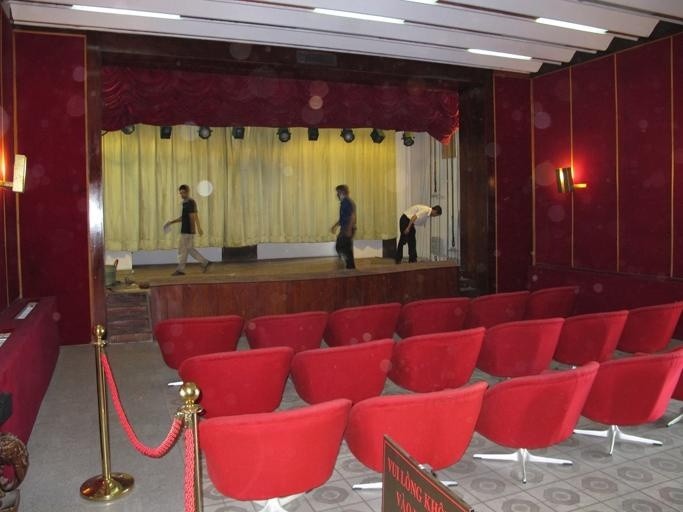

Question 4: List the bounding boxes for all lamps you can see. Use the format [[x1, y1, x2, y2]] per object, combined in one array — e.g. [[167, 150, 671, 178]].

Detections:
[[0, 154, 27, 192], [121, 124, 414, 147], [556, 166, 587, 194]]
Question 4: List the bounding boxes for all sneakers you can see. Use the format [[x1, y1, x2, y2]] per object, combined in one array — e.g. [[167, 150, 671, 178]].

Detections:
[[171, 271, 186, 276], [201, 261, 212, 272]]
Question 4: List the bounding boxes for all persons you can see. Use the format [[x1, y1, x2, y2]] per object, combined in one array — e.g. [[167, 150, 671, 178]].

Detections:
[[331, 185, 357, 271], [395, 205, 442, 264], [163, 185, 211, 276]]
